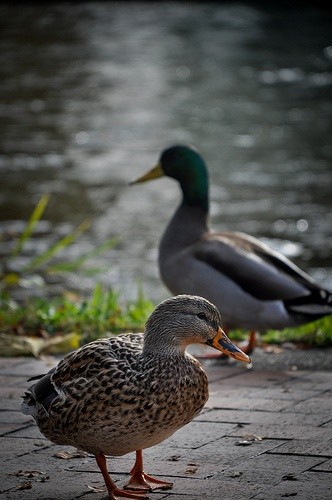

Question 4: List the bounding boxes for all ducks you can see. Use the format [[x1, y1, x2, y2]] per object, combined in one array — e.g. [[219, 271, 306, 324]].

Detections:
[[127, 145, 332, 360], [16, 294, 251, 499]]
